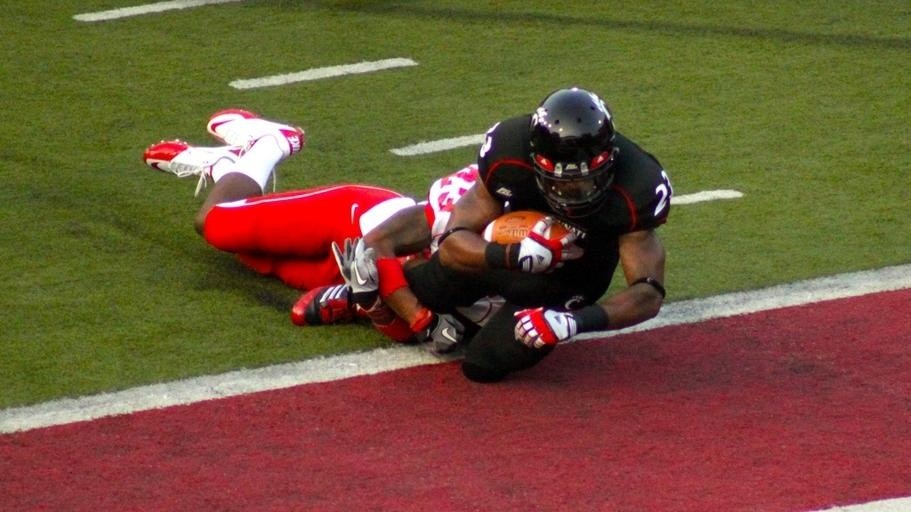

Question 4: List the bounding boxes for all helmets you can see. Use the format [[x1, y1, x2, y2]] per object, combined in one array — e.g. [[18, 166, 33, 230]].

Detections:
[[530, 88, 617, 219]]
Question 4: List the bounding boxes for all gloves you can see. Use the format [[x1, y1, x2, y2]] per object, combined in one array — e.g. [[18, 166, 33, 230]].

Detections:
[[514, 303, 609, 350], [411, 312, 463, 355], [487, 215, 584, 276], [331, 238, 382, 312]]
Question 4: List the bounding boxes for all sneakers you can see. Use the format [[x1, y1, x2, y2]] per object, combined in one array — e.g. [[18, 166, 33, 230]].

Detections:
[[206, 108, 304, 159], [144, 140, 246, 178], [291, 286, 361, 326]]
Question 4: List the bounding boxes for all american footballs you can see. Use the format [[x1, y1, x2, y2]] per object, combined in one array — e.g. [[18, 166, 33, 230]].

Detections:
[[481, 210, 573, 248]]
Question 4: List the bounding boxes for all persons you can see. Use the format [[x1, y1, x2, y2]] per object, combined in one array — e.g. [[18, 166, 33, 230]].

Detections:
[[143, 108, 504, 358], [291, 87, 673, 383]]
